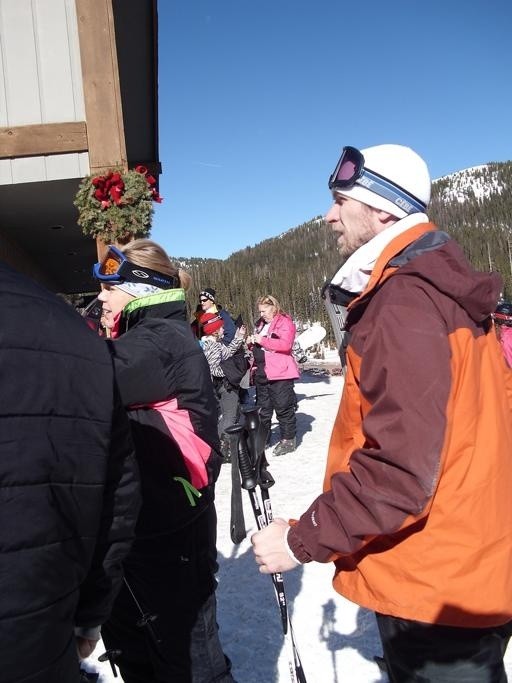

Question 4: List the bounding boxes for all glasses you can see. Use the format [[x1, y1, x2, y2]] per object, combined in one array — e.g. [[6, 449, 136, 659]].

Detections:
[[94, 245, 129, 284], [329, 147, 364, 187]]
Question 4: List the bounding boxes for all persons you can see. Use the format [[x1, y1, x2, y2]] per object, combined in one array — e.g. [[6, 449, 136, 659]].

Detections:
[[250, 142, 512, 683], [493, 301, 512, 368], [0, 238, 302, 682]]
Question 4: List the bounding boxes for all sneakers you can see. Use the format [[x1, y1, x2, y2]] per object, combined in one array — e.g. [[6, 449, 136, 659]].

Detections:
[[272, 436, 297, 456]]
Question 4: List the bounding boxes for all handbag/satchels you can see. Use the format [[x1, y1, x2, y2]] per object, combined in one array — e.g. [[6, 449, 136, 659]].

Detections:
[[219, 356, 250, 387]]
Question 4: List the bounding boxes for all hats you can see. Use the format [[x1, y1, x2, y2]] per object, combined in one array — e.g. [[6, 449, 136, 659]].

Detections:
[[194, 311, 224, 335], [199, 288, 216, 304], [331, 144, 431, 219]]
